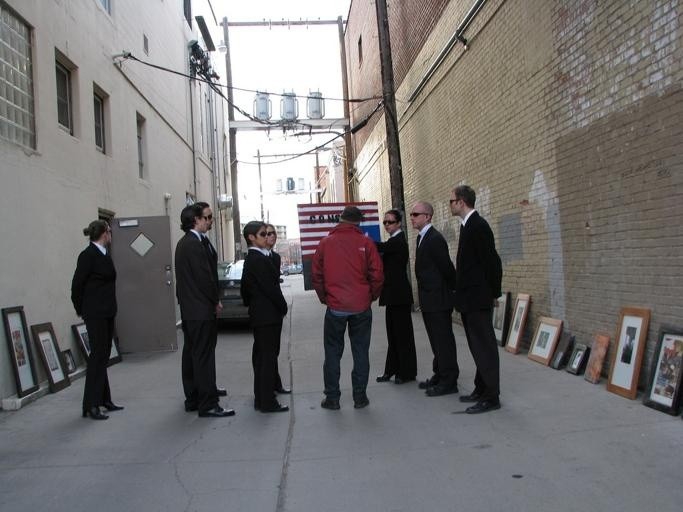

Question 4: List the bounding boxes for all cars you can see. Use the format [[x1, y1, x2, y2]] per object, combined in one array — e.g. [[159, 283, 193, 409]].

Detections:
[[281, 265, 303, 276], [203, 260, 285, 319]]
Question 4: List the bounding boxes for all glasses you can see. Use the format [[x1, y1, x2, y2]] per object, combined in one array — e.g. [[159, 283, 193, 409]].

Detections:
[[107, 230, 112, 234], [267, 231, 276, 236], [202, 216, 207, 220], [450, 200, 459, 204], [383, 220, 396, 224], [410, 212, 426, 216], [260, 232, 268, 236], [208, 213, 212, 220]]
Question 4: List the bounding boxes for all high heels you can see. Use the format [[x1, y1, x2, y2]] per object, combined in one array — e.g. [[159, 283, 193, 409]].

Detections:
[[98, 397, 123, 410], [394, 374, 416, 383], [376, 373, 398, 382], [82, 406, 109, 420]]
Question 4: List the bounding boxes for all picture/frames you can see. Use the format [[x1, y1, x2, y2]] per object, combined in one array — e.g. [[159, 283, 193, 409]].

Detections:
[[492, 292, 683, 418], [30, 321, 123, 393], [1, 305, 40, 398]]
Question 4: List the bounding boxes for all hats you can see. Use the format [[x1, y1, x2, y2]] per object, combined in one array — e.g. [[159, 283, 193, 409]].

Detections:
[[341, 206, 364, 221]]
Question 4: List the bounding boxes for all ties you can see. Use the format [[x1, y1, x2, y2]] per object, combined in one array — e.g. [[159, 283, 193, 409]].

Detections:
[[416, 234, 422, 253], [105, 248, 110, 258]]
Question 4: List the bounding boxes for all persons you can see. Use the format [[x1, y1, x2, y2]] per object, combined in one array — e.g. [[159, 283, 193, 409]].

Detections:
[[313, 208, 385, 410], [263, 223, 293, 395], [410, 202, 460, 395], [374, 209, 417, 384], [196, 200, 227, 397], [71, 219, 124, 419], [175, 206, 235, 416], [449, 185, 504, 413], [241, 221, 290, 412]]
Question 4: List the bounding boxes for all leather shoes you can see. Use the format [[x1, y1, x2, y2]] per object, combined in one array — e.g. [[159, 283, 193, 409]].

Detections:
[[419, 377, 438, 389], [217, 389, 226, 396], [466, 400, 500, 414], [185, 405, 199, 412], [425, 385, 458, 396], [354, 399, 369, 408], [199, 404, 235, 416], [277, 386, 291, 393], [321, 399, 340, 409], [255, 401, 288, 412], [459, 387, 483, 402]]
